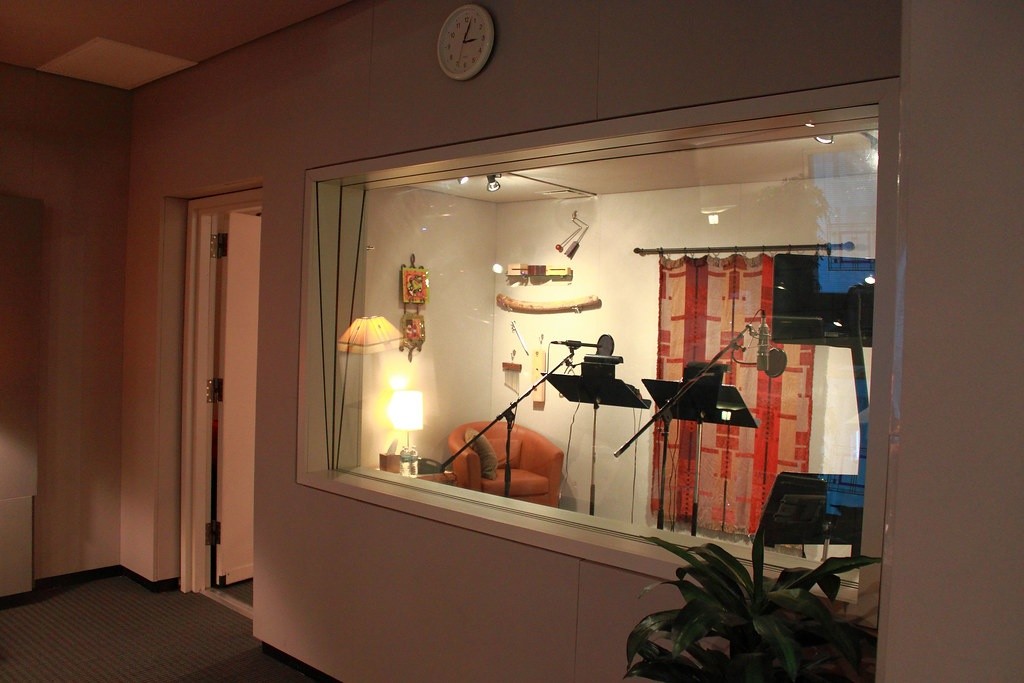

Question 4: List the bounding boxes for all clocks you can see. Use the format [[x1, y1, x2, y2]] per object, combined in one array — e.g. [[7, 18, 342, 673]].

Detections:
[[437, 5, 494, 80]]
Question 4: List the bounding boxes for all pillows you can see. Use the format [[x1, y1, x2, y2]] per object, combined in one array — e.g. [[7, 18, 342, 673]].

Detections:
[[488, 439, 522, 469], [465, 428, 498, 480]]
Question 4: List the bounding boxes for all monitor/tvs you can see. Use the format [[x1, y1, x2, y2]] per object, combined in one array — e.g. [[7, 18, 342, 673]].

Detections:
[[754, 469, 863, 547], [769, 251, 874, 347]]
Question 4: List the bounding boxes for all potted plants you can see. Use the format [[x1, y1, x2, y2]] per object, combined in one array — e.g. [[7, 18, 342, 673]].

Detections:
[[621, 534, 883, 683]]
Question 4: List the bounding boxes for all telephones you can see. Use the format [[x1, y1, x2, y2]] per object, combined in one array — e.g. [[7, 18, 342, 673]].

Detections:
[[418, 457, 445, 474]]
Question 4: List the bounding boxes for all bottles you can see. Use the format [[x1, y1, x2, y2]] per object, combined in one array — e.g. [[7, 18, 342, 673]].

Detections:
[[400, 446, 418, 478]]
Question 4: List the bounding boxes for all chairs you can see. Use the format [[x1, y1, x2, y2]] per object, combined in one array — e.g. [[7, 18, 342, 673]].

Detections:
[[449, 422, 564, 507]]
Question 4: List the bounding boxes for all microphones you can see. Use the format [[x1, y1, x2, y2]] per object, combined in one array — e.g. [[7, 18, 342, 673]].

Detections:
[[755, 317, 787, 377], [566, 333, 614, 357]]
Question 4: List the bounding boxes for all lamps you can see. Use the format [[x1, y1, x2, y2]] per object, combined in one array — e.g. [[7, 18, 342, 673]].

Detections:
[[814, 135, 833, 145], [457, 176, 468, 184], [487, 174, 502, 192]]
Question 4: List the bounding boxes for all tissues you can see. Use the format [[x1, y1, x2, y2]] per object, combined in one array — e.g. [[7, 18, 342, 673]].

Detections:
[[379, 439, 401, 474]]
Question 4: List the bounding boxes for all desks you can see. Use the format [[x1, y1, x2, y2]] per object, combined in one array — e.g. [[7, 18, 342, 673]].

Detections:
[[400, 469, 455, 486]]
